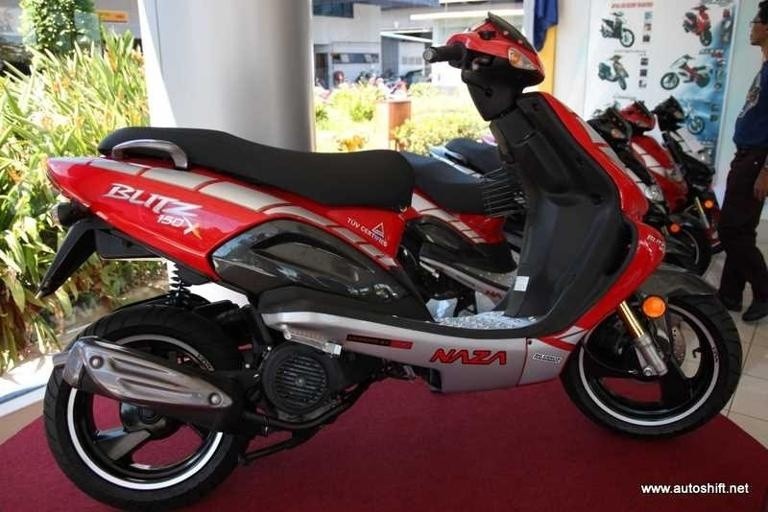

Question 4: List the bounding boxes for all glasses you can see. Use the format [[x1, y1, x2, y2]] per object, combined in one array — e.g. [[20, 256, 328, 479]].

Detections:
[[749, 21, 763, 28]]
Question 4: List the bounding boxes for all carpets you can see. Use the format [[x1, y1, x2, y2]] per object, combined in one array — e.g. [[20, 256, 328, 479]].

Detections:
[[0, 376, 768, 511]]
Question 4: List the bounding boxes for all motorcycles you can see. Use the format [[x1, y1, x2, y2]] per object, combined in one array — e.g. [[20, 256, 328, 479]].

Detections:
[[40, 9, 742, 512], [589, 3, 737, 135], [394, 92, 726, 312]]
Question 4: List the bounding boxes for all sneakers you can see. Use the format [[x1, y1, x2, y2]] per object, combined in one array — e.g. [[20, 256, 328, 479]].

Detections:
[[719, 295, 743, 312], [742, 300, 768, 321]]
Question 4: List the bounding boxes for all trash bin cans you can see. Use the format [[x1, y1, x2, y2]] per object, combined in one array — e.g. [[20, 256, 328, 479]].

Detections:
[[377, 98, 412, 152]]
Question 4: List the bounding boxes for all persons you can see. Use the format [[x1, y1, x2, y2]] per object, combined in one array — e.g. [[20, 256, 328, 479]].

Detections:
[[714, 0, 768, 322]]
